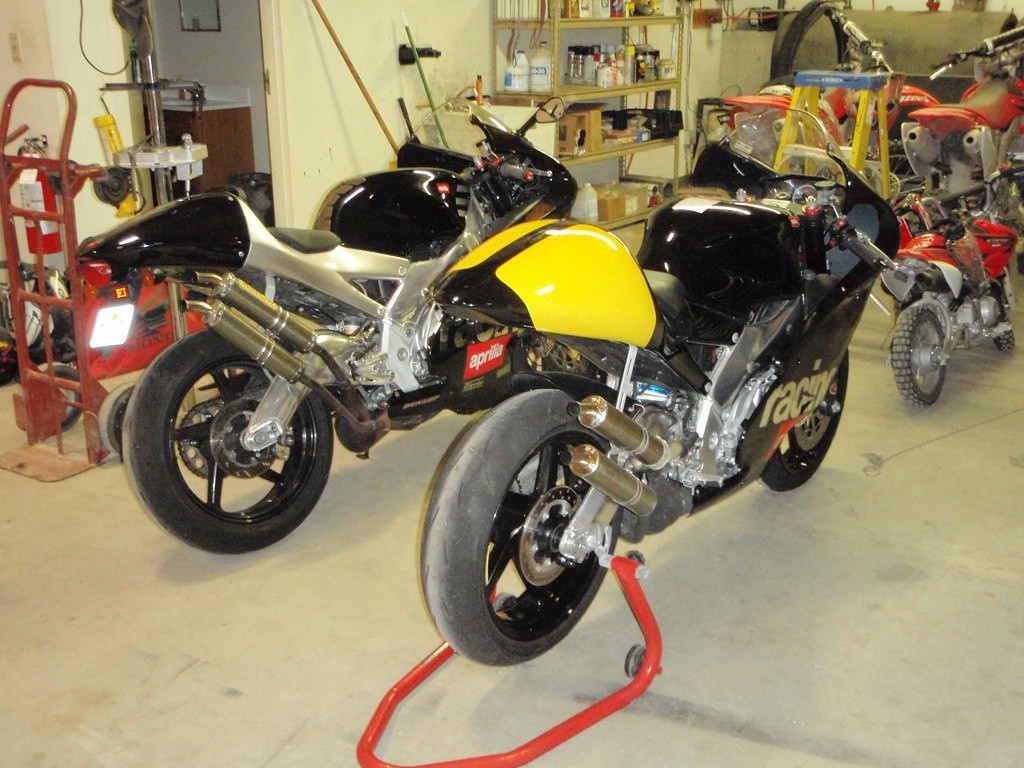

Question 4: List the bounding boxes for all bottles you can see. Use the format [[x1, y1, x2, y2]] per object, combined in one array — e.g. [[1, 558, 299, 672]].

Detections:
[[567, 44, 637, 87], [571, 182, 599, 225], [503, 50, 530, 93], [529, 41, 553, 94]]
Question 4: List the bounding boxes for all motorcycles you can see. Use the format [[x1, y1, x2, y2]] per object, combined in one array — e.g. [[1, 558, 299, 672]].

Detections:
[[681, 5, 1024, 411], [411, 95, 924, 669], [69, 93, 610, 557]]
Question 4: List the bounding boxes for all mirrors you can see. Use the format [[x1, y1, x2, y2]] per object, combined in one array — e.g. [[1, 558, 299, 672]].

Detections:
[[179, 0, 221, 32]]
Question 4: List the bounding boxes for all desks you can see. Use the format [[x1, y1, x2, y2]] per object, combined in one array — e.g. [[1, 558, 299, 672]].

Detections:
[[163, 107, 255, 201]]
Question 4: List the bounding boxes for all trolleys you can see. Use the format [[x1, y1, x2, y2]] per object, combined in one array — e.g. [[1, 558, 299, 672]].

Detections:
[[0, 75, 139, 486]]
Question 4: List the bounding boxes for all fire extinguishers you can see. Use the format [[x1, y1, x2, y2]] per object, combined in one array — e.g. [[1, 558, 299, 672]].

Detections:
[[16, 134, 63, 255]]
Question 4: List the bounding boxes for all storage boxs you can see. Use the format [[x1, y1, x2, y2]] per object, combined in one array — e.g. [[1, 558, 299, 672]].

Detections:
[[634, 130, 651, 142], [593, 183, 626, 220], [559, 102, 609, 154], [492, 0, 684, 230], [603, 133, 635, 145], [624, 186, 648, 215], [613, 108, 684, 140]]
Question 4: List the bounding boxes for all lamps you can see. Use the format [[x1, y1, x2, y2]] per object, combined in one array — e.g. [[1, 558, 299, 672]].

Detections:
[[708, 14, 723, 40]]
